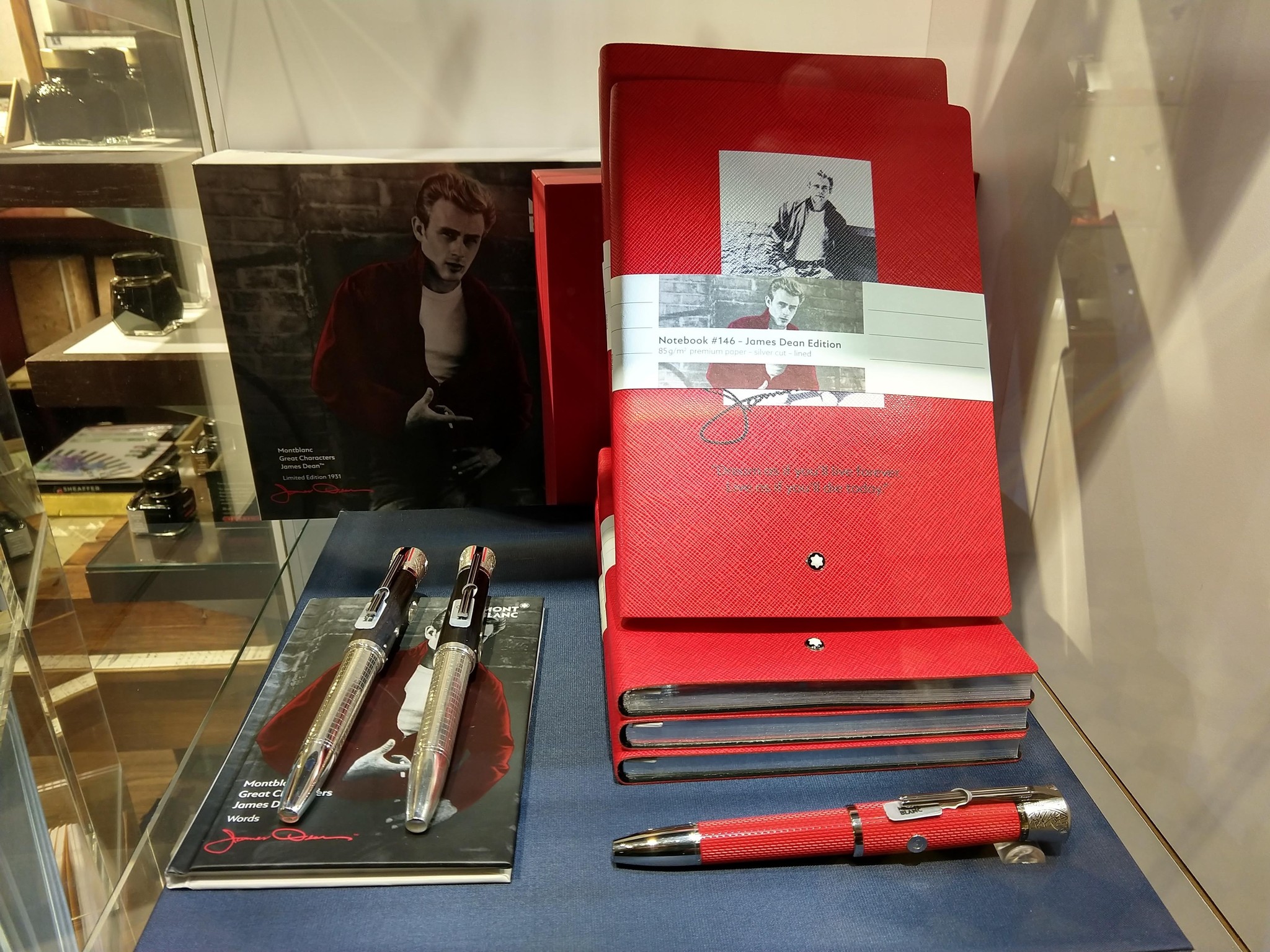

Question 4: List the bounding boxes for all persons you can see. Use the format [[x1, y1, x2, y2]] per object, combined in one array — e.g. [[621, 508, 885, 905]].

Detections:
[[310, 169, 541, 513], [768, 168, 850, 278], [707, 276, 819, 390]]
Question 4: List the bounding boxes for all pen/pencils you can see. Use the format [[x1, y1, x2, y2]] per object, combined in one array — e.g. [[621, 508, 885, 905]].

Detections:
[[277, 545, 429, 822], [405, 546, 497, 832], [610, 784, 1072, 868]]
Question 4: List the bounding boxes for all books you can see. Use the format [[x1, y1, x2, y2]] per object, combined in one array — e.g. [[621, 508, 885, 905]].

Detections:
[[598, 41, 949, 409], [609, 76, 1016, 622], [595, 445, 1040, 787]]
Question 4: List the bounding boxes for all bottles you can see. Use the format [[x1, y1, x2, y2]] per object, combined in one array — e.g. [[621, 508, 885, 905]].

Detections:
[[25, 67, 131, 147], [110, 251, 184, 334], [0, 509, 35, 562], [126, 465, 198, 538], [51, 48, 155, 138]]
